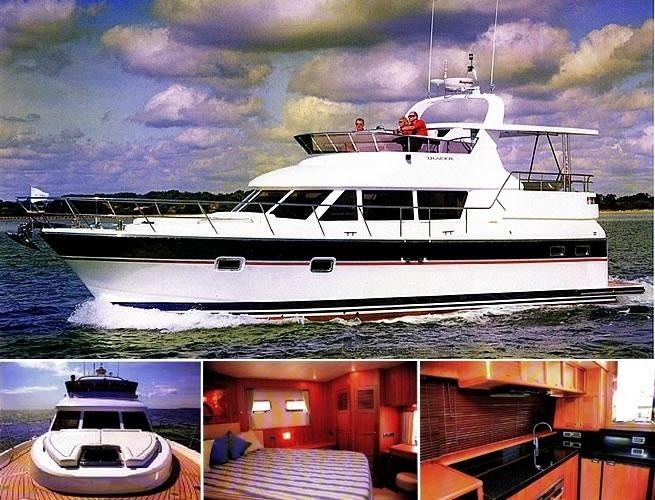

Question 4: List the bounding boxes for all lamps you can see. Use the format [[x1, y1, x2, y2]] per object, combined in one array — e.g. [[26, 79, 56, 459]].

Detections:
[[252, 399, 272, 415], [285, 400, 304, 411]]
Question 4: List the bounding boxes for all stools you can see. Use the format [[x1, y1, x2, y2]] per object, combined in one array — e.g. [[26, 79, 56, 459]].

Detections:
[[394, 469, 417, 492]]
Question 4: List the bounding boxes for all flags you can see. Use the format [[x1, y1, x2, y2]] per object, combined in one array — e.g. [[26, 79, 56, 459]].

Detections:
[[29, 186, 49, 204]]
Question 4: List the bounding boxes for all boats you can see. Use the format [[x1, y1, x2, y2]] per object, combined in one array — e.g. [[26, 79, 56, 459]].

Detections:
[[0, 363, 202, 500], [1, 2, 644, 324]]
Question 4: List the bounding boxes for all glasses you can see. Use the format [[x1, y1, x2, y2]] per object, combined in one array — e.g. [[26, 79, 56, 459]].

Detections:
[[408, 115, 415, 117], [399, 121, 402, 123]]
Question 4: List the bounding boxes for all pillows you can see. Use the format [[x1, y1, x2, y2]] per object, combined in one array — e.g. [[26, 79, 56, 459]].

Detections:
[[227, 429, 253, 461], [237, 427, 263, 455], [209, 436, 229, 467], [204, 440, 215, 473]]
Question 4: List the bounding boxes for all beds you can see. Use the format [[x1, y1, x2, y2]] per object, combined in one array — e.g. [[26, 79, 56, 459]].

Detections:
[[204, 421, 375, 499]]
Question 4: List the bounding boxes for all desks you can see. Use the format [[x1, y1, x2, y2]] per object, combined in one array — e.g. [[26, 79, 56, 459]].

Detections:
[[421, 460, 485, 500], [390, 442, 417, 463]]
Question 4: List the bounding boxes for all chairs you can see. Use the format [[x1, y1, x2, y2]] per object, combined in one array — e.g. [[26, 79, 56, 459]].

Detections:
[[350, 127, 468, 153]]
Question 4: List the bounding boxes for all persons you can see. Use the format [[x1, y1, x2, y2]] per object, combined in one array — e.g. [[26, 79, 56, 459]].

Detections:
[[351, 117, 368, 132], [397, 111, 429, 136], [396, 117, 415, 136]]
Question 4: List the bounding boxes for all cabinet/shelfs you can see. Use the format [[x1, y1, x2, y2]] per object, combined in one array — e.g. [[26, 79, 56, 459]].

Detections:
[[380, 362, 417, 407], [553, 368, 605, 430], [552, 361, 588, 397], [523, 361, 565, 386], [459, 358, 522, 391], [579, 451, 655, 500], [564, 453, 579, 500]]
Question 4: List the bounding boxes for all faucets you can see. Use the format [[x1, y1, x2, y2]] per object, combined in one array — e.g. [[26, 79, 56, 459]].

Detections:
[[533, 448, 554, 471], [532, 421, 553, 448]]
